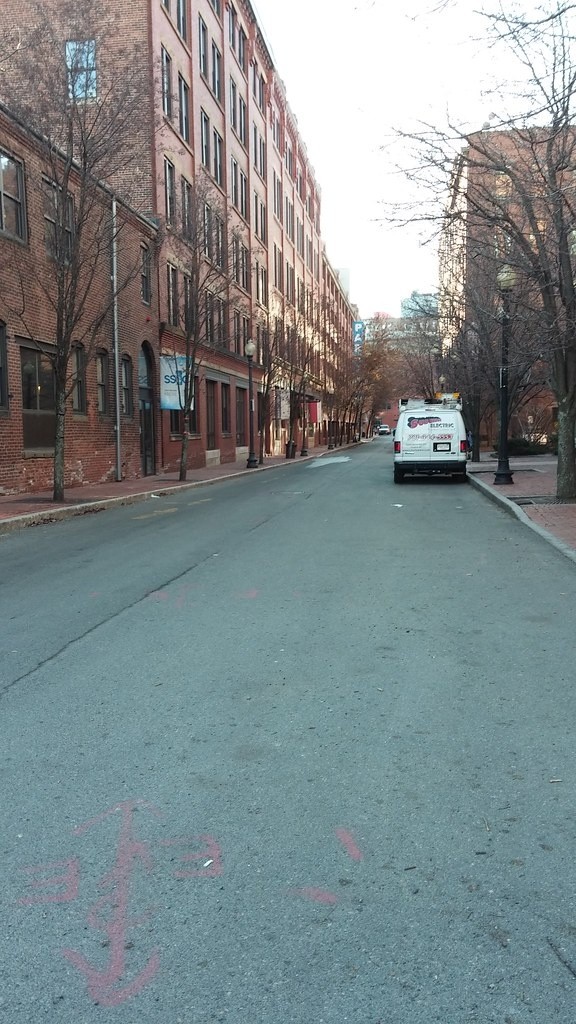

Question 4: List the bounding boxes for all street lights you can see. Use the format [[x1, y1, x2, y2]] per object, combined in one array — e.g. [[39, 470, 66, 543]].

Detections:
[[245, 337, 258, 468], [328, 385, 335, 450], [439, 375, 447, 393], [493, 264, 514, 485]]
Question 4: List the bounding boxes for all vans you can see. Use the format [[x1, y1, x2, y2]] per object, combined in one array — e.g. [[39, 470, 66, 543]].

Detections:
[[393, 392, 470, 484]]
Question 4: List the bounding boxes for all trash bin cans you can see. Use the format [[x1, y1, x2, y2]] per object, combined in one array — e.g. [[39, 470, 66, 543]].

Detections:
[[285, 440, 297, 459]]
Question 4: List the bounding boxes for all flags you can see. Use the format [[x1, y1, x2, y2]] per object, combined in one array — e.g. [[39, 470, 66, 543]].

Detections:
[[159, 355, 194, 410], [307, 401, 321, 423]]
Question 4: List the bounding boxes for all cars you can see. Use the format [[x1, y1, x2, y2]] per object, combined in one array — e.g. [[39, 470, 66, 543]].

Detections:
[[379, 425, 390, 435]]
